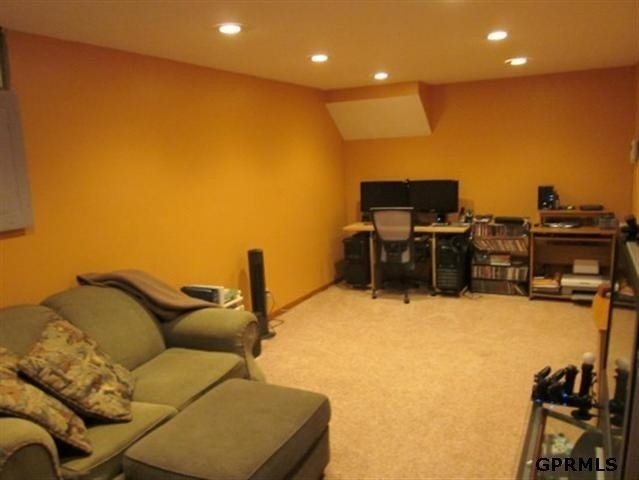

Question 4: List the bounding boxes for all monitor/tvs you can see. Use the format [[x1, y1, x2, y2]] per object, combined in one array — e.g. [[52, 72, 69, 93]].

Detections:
[[361, 182, 406, 212], [406, 181, 458, 226]]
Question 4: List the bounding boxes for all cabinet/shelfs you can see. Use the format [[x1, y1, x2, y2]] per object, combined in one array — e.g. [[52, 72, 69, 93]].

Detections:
[[526, 208, 620, 310], [468, 214, 530, 298]]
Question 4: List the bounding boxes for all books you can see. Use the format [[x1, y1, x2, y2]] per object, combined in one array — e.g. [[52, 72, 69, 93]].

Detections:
[[533, 275, 561, 295], [472, 222, 529, 295]]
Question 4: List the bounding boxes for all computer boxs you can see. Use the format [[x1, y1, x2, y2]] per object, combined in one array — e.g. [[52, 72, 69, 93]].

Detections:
[[437, 245, 466, 290], [343, 233, 371, 284]]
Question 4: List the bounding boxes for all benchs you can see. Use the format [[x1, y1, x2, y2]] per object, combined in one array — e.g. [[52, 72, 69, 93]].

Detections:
[[122, 375, 332, 479]]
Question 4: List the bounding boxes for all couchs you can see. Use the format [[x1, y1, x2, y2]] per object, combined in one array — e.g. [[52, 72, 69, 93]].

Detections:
[[0, 265, 266, 479]]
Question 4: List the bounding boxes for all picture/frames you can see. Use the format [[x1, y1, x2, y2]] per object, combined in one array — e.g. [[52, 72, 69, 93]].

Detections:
[[0, 90, 35, 235]]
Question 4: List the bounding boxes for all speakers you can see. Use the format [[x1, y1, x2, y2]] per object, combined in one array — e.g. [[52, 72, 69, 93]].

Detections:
[[248, 248, 276, 338], [539, 187, 553, 209]]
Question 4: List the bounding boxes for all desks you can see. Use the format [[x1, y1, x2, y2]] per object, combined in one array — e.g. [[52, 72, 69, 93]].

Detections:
[[514, 388, 605, 478], [341, 220, 472, 298]]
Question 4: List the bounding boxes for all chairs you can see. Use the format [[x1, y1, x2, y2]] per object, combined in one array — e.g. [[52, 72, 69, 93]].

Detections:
[[369, 206, 431, 305]]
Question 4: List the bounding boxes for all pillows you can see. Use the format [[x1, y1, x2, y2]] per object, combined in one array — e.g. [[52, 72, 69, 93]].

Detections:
[[14, 316, 139, 423], [0, 344, 94, 456]]
